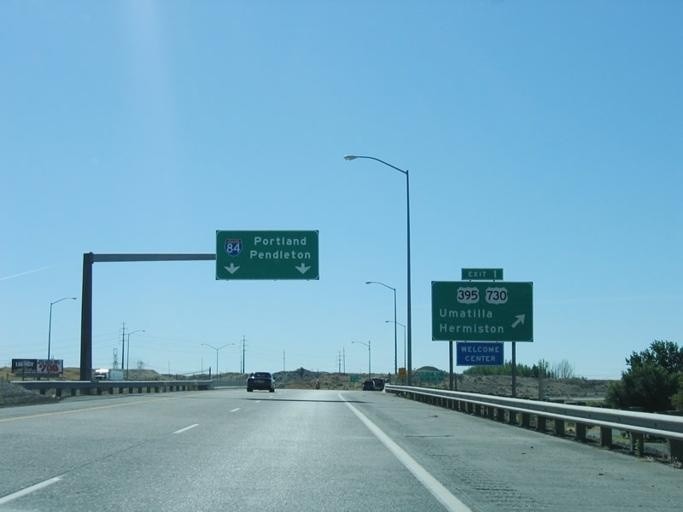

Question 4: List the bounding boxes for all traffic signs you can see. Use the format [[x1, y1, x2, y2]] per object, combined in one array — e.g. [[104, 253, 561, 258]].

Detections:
[[457, 342, 504, 365], [432, 281, 533, 341], [216, 231, 319, 279]]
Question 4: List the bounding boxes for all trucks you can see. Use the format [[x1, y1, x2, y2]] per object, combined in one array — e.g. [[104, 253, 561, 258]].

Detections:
[[95, 368, 123, 381]]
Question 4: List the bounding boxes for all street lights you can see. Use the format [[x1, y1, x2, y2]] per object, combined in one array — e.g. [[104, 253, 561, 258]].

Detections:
[[121, 320, 146, 380], [48, 297, 77, 360], [203, 342, 235, 384], [344, 155, 411, 392]]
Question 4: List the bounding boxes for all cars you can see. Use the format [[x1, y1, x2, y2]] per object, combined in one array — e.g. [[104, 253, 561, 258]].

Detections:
[[246, 372, 276, 392]]
